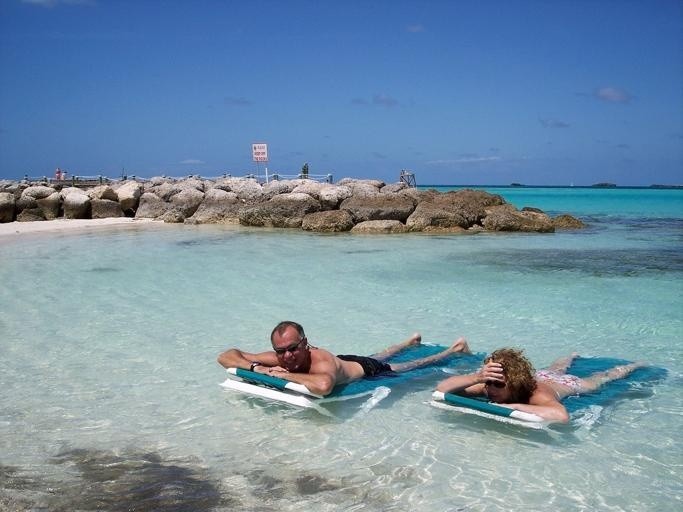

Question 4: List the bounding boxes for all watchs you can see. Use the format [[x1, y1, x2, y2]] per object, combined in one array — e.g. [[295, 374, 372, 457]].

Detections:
[[249, 360, 263, 372]]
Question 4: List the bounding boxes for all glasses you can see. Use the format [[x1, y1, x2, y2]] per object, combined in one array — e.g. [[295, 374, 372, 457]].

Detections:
[[277, 337, 304, 354]]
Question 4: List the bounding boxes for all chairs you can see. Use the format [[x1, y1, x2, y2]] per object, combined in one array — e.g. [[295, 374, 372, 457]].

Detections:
[[219, 343, 496, 426], [419, 354, 667, 447]]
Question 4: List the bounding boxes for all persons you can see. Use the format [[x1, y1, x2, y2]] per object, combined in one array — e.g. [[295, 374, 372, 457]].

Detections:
[[433, 344, 651, 425], [217, 320, 469, 398]]
